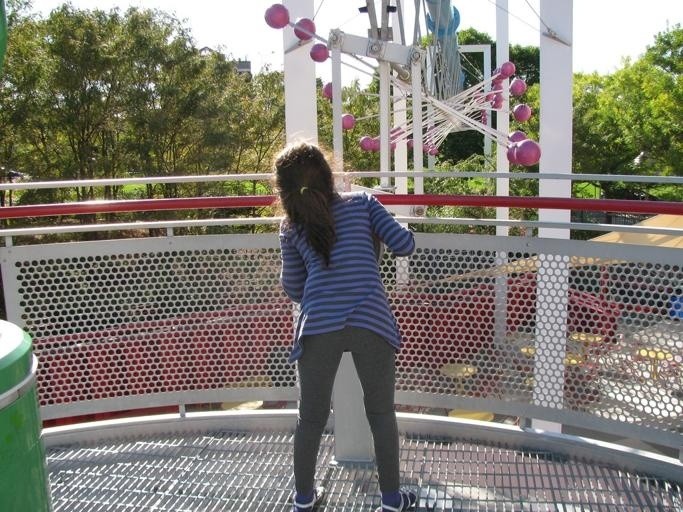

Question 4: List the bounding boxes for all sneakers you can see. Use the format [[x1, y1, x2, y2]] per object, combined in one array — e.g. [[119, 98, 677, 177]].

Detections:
[[291, 486, 325, 512], [380, 490, 419, 512]]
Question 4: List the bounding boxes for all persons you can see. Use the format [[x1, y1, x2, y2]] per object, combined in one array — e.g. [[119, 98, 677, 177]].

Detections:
[[273, 143, 415, 512]]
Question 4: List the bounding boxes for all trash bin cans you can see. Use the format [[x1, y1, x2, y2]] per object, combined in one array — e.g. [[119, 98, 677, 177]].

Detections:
[[0, 318, 53, 512]]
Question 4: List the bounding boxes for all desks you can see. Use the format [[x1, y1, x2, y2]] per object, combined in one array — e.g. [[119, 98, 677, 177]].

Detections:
[[219, 329, 676, 424]]
